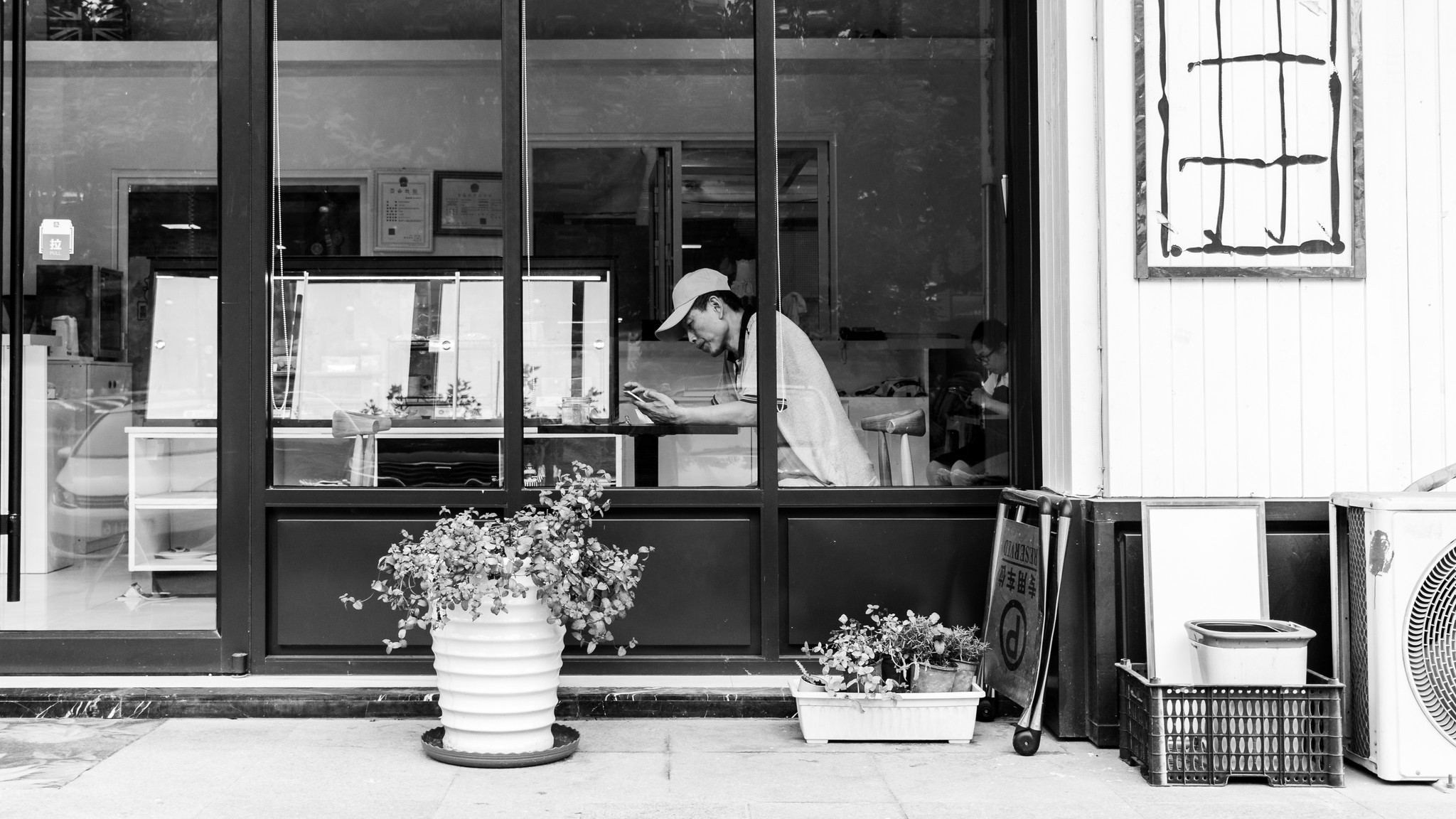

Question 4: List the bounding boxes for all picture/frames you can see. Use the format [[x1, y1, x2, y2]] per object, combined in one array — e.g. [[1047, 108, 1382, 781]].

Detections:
[[1124, 0, 1374, 287]]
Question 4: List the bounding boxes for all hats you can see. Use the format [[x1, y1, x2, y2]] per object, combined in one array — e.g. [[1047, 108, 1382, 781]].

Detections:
[[655, 268, 730, 342]]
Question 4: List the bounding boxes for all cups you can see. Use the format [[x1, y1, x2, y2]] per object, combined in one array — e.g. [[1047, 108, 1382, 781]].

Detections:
[[421, 415, 432, 420], [562, 397, 590, 425]]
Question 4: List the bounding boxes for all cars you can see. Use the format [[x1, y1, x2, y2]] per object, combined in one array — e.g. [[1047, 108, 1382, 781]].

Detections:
[[47, 400, 196, 555]]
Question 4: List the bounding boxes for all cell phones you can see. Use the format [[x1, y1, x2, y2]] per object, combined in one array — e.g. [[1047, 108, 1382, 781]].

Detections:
[[623, 391, 646, 404]]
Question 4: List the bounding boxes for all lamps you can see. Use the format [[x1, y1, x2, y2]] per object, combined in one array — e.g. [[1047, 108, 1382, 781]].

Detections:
[[159, 190, 203, 236]]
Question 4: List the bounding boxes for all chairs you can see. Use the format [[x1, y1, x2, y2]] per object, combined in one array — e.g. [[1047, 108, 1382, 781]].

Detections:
[[943, 412, 1013, 479], [856, 408, 927, 486], [332, 409, 392, 488]]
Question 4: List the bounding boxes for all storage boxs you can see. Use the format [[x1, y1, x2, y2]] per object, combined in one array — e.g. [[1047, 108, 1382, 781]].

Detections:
[[1112, 657, 1345, 790]]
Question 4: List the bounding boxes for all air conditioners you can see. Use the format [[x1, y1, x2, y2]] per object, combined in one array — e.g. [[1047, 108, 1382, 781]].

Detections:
[[1325, 487, 1456, 787]]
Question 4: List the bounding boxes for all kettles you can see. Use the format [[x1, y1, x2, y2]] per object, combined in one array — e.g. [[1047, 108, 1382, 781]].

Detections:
[[49, 315, 79, 357]]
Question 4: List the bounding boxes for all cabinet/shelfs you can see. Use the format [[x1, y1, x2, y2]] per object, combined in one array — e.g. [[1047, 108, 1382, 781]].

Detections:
[[45, 357, 136, 557], [122, 423, 636, 599]]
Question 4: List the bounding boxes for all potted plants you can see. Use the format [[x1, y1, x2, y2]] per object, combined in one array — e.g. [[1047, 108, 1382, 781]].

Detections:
[[789, 600, 993, 704], [338, 460, 660, 768]]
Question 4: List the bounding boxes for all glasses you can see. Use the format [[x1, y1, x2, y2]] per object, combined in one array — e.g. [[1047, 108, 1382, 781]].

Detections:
[[974, 344, 999, 363]]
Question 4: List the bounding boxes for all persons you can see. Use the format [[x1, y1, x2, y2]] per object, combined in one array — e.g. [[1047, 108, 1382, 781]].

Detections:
[[923, 321, 1011, 486], [622, 268, 882, 489]]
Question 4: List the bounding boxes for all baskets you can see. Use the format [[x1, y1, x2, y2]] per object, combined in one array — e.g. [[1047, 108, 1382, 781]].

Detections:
[[1114, 660, 1343, 786]]
